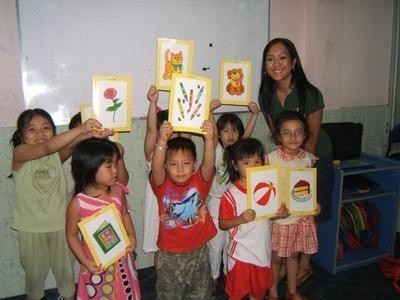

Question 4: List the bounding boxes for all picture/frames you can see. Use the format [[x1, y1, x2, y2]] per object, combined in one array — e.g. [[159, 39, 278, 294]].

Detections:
[[282, 167, 315, 216], [155, 37, 193, 91], [80, 102, 120, 143], [166, 74, 212, 136], [91, 75, 133, 135], [245, 166, 284, 223], [76, 201, 130, 275], [217, 61, 252, 107]]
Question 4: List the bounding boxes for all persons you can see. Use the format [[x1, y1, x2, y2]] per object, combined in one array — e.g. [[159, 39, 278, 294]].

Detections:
[[6, 109, 115, 300], [257, 38, 334, 288], [149, 120, 218, 300], [66, 137, 142, 299], [143, 85, 191, 282], [218, 138, 287, 299], [264, 110, 321, 300], [68, 112, 129, 186], [206, 99, 259, 300]]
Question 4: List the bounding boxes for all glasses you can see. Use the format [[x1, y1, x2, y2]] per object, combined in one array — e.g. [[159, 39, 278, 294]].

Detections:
[[278, 129, 308, 139]]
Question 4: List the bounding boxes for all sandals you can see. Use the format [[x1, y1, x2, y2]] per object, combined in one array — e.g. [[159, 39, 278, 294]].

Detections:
[[284, 290, 306, 300], [277, 256, 286, 284], [296, 265, 315, 288]]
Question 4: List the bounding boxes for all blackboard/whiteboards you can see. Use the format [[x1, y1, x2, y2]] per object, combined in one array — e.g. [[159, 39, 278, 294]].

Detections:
[[16, 0, 270, 129]]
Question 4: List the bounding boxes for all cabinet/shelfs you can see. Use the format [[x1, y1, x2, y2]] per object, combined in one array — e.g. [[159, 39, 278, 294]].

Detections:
[[311, 152, 400, 276]]
